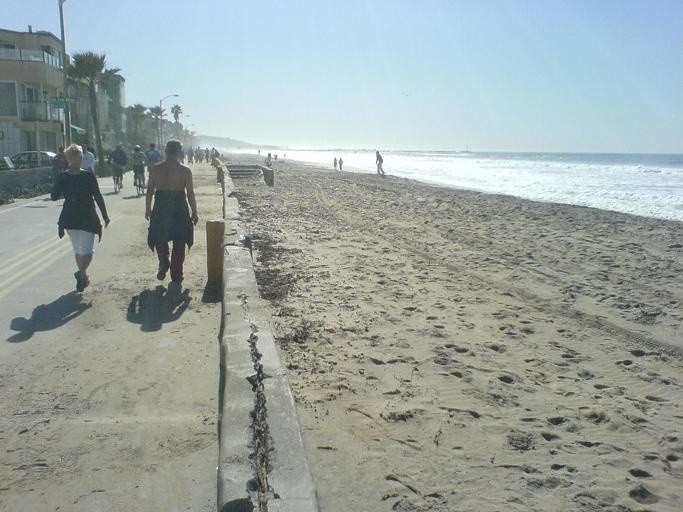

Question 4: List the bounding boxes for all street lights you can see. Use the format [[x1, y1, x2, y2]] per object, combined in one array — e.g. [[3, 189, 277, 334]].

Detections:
[[159, 94, 180, 145]]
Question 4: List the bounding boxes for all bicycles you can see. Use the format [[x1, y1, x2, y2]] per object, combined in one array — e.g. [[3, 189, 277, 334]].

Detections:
[[113, 168, 123, 193], [136, 164, 146, 196]]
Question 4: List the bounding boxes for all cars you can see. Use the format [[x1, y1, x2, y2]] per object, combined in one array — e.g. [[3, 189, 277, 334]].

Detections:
[[0, 151, 57, 170]]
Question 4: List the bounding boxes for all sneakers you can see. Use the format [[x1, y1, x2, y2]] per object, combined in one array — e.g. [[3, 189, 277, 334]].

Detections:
[[74, 270, 89, 291], [170, 267, 183, 280], [156, 262, 170, 281]]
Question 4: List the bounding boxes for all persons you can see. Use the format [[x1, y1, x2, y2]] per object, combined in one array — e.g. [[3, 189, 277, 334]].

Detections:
[[264, 153, 270, 167], [334, 157, 343, 170], [53, 145, 219, 188], [375, 151, 385, 175], [145, 141, 197, 281], [50, 144, 109, 292]]
